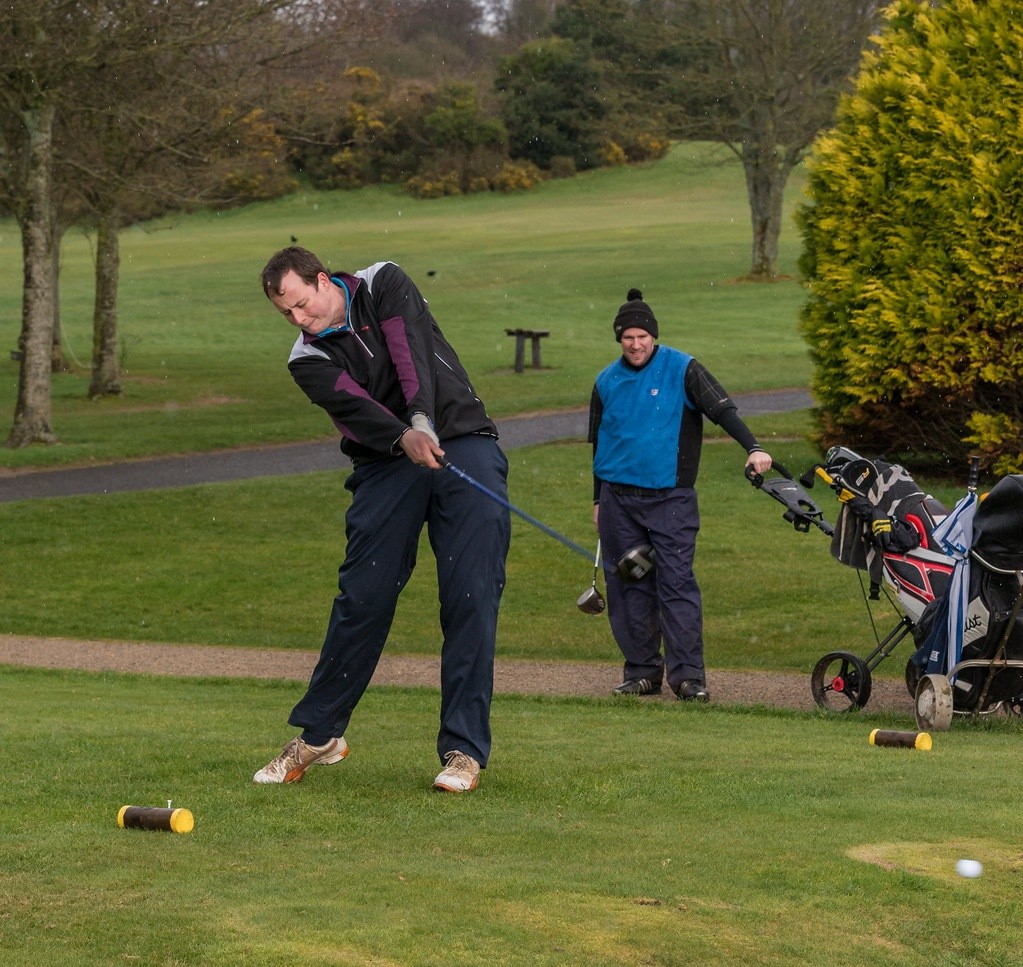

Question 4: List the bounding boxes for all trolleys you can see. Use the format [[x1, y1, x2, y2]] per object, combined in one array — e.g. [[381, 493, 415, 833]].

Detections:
[[898, 455, 1023, 739], [742, 448, 952, 721]]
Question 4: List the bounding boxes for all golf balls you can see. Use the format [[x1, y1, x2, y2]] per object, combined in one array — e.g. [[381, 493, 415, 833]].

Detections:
[[956, 859, 982, 879]]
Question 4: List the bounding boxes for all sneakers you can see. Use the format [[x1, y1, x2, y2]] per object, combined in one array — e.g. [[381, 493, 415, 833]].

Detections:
[[611, 677, 662, 695], [676, 680, 710, 702], [434, 749, 481, 793], [252, 735, 349, 786]]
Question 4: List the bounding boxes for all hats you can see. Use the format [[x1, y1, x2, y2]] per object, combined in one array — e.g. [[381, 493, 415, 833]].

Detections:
[[614, 288, 658, 343]]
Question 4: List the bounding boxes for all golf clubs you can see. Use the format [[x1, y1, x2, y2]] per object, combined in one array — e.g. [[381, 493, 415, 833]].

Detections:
[[576, 535, 606, 615], [429, 449, 657, 578]]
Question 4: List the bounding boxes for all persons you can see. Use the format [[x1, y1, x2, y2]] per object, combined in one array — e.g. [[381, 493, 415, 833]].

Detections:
[[588, 289, 773, 703], [253, 246, 512, 791]]
